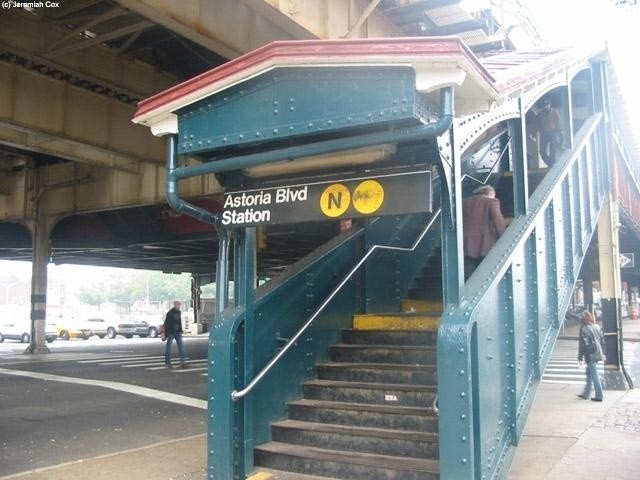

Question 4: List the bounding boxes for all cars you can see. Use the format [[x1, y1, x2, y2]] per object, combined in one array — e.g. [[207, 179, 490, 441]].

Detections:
[[0, 313, 161, 343]]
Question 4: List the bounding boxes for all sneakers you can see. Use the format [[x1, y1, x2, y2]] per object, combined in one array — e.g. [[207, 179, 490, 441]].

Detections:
[[576, 394, 602, 401]]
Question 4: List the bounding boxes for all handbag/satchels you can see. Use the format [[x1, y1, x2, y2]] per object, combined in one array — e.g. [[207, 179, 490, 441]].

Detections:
[[582, 337, 596, 355], [159, 325, 167, 341]]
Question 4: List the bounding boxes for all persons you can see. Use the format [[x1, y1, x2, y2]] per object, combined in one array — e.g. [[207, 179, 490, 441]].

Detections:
[[533, 98, 563, 168], [164, 301, 189, 370], [575, 313, 605, 401], [462, 185, 506, 280]]
